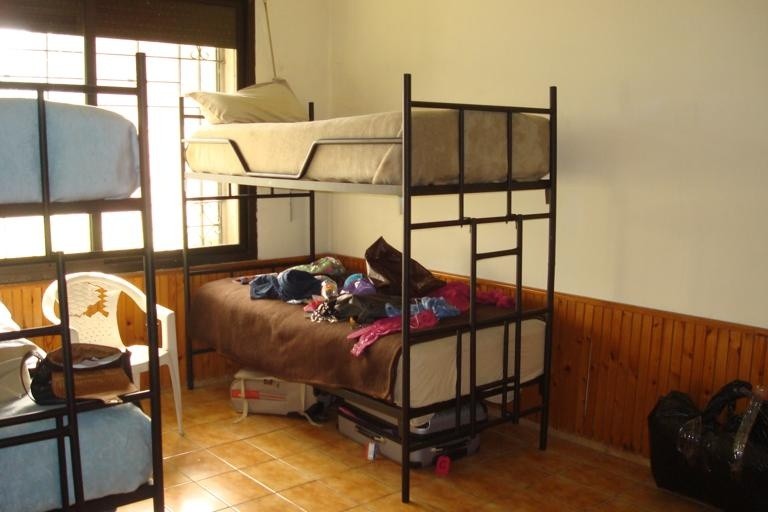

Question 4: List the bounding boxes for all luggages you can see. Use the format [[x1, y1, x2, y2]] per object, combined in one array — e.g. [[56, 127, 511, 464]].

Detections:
[[333, 396, 487, 469]]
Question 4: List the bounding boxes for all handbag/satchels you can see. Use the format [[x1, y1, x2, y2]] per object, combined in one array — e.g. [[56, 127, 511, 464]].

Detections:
[[31, 343, 139, 406], [230, 378, 344, 423], [648, 389, 768, 511]]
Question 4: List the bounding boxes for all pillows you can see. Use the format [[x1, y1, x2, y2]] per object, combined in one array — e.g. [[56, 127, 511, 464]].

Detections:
[[184, 73, 311, 125]]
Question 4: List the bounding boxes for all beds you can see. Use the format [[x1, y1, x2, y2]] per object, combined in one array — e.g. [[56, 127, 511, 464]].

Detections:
[[179, 73, 557, 503], [0, 52, 165, 512]]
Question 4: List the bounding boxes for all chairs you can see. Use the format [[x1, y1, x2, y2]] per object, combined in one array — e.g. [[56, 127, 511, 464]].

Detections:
[[41, 271, 185, 435]]
